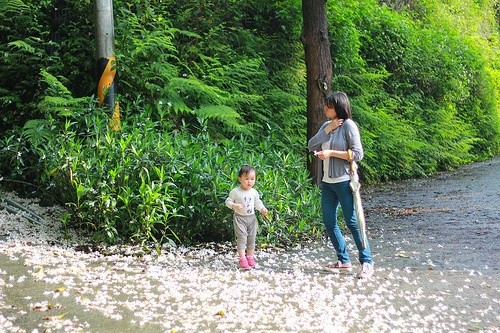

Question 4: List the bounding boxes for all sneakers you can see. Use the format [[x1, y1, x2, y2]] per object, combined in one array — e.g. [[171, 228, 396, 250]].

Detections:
[[324, 260, 352, 271], [357, 262, 374, 277], [239, 256, 250, 269], [247, 255, 255, 267]]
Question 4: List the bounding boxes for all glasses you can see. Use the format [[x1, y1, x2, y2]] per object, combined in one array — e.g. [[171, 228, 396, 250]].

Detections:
[[327, 102, 334, 109]]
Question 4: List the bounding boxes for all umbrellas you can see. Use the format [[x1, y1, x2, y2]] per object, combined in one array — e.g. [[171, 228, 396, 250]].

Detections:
[[347, 148, 368, 249]]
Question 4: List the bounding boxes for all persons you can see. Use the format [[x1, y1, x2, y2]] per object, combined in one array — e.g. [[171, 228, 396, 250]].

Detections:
[[308, 92, 375, 279], [225, 166, 268, 270]]
